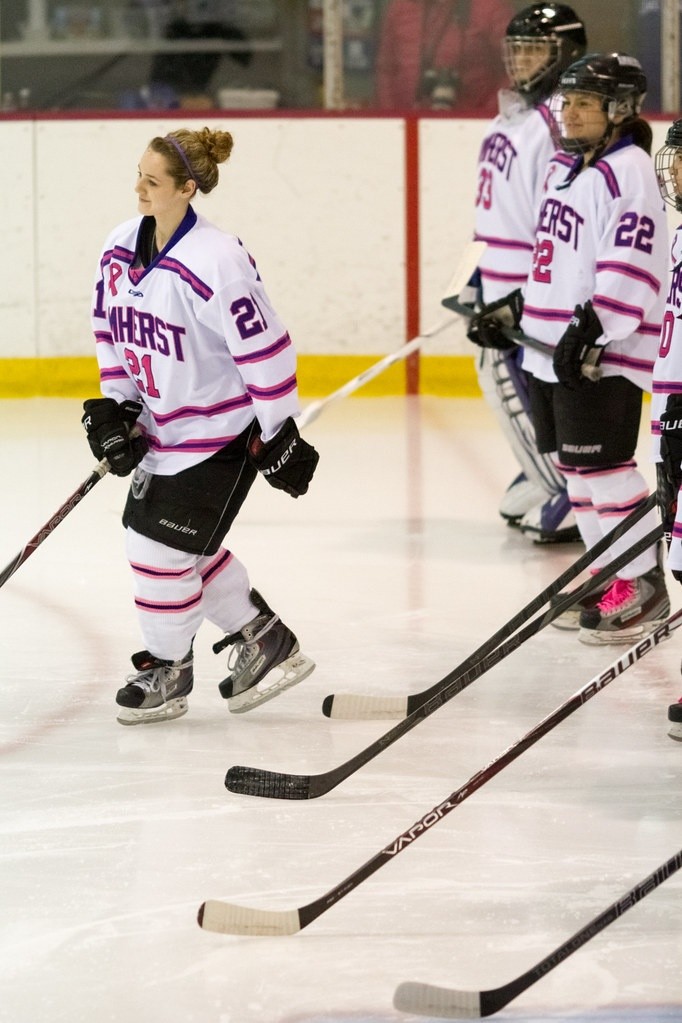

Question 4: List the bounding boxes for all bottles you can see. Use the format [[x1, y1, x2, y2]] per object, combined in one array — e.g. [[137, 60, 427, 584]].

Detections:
[[0, 90, 19, 114], [19, 88, 31, 109]]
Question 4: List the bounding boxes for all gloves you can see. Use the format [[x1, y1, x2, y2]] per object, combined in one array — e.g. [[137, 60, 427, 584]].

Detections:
[[250, 417, 319, 499], [466, 289, 525, 350], [79, 396, 152, 476], [554, 300, 608, 385]]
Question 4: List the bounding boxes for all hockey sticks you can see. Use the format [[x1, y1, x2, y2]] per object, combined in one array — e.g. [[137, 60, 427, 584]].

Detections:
[[0, 427, 143, 589], [293, 311, 468, 426], [224, 521, 675, 801], [392, 850, 682, 1022], [322, 492, 660, 721], [442, 296, 603, 382], [196, 607, 682, 939]]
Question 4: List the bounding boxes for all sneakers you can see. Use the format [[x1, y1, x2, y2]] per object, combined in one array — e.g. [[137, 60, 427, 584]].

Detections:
[[547, 568, 672, 646], [113, 650, 195, 725], [498, 474, 583, 547], [217, 614, 314, 713]]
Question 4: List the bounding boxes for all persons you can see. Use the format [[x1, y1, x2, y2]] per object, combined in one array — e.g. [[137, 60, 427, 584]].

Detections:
[[82, 127, 320, 709], [375, 0, 518, 109], [466, 2, 682, 722]]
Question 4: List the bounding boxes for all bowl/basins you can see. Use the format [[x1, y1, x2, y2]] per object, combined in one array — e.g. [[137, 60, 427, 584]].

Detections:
[[216, 88, 279, 110]]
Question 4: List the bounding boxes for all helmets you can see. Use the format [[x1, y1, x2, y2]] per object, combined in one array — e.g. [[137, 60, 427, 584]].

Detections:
[[547, 52, 647, 154], [503, 1, 588, 109], [655, 120, 682, 212]]
